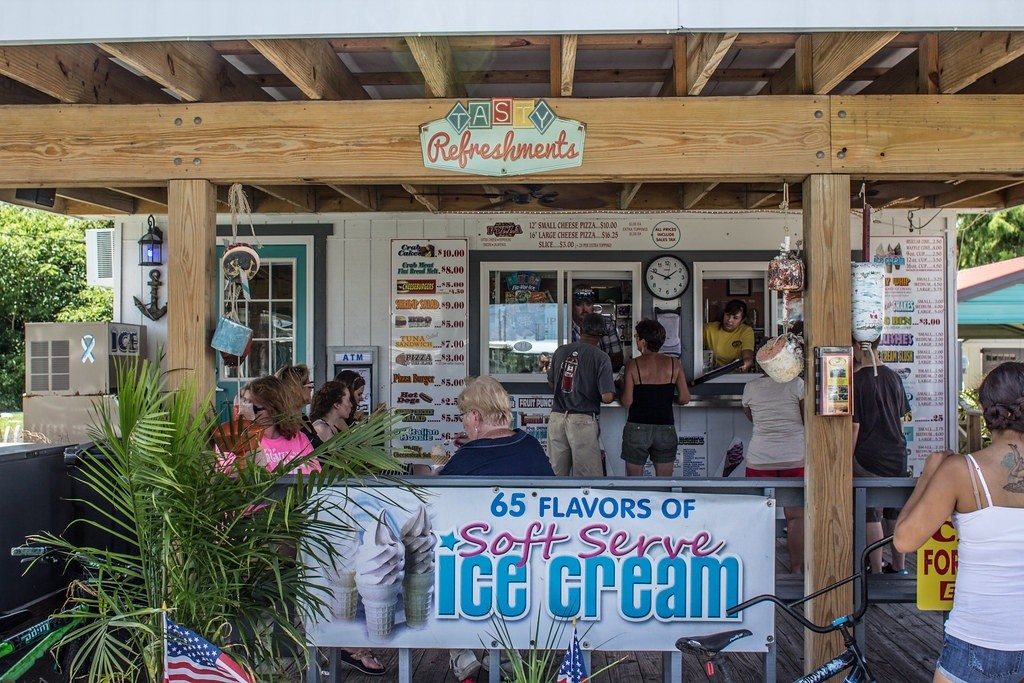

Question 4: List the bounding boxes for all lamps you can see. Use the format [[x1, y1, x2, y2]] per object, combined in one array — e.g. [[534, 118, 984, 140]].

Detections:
[[137, 214, 164, 266]]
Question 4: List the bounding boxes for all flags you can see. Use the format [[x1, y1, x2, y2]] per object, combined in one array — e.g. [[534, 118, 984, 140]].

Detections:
[[164, 613, 250, 683]]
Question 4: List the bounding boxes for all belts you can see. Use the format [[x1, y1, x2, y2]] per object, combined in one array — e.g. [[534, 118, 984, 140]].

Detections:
[[551, 410, 599, 416]]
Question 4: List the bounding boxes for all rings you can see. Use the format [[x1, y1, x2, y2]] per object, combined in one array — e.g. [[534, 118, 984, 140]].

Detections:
[[464, 432, 466, 437]]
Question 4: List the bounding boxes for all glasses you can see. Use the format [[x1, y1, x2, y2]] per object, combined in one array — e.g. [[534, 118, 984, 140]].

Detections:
[[458, 409, 473, 422], [252, 405, 266, 414], [302, 381, 314, 389], [573, 299, 594, 307], [352, 375, 364, 386], [634, 334, 641, 342]]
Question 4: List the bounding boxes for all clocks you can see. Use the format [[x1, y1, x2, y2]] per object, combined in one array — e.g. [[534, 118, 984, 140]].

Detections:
[[644, 254, 690, 300]]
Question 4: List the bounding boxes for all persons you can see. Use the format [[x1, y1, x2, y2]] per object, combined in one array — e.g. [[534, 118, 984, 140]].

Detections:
[[213, 364, 368, 544], [741, 371, 806, 576], [703, 300, 755, 374], [851, 334, 911, 576], [616, 319, 690, 477], [435, 377, 557, 477], [544, 312, 616, 477], [571, 286, 624, 372], [892, 361, 1024, 683]]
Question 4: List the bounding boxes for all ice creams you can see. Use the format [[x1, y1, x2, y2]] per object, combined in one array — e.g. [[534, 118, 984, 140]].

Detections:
[[876, 242, 902, 273], [723, 442, 744, 478], [400, 505, 438, 630], [355, 508, 405, 642], [327, 510, 360, 620], [432, 443, 449, 465]]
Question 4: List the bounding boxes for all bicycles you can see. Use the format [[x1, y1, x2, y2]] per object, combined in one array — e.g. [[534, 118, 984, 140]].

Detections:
[[674, 534, 896, 683]]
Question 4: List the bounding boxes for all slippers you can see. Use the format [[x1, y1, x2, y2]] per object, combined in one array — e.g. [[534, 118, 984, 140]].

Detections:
[[341, 649, 386, 675]]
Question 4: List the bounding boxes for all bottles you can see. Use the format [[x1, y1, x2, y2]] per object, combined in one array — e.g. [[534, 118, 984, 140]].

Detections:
[[526, 427, 548, 439], [720, 301, 730, 322], [510, 396, 515, 408], [738, 298, 746, 304], [851, 263, 886, 350], [745, 299, 758, 328], [224, 364, 234, 378], [708, 296, 719, 322]]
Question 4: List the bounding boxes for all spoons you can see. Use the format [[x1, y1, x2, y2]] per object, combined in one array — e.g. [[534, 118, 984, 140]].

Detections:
[[443, 438, 455, 446]]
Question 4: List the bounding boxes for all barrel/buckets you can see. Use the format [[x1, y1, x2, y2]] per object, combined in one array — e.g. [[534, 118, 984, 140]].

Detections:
[[210, 316, 253, 357]]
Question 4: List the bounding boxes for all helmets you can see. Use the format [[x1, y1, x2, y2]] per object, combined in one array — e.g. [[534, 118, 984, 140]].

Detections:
[[573, 285, 594, 298]]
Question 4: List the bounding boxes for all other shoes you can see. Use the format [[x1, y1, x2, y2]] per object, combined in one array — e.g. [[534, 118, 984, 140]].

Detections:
[[884, 563, 907, 574]]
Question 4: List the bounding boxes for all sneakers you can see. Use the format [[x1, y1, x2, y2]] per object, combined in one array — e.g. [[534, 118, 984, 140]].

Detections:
[[450, 649, 482, 681], [482, 653, 517, 681]]
